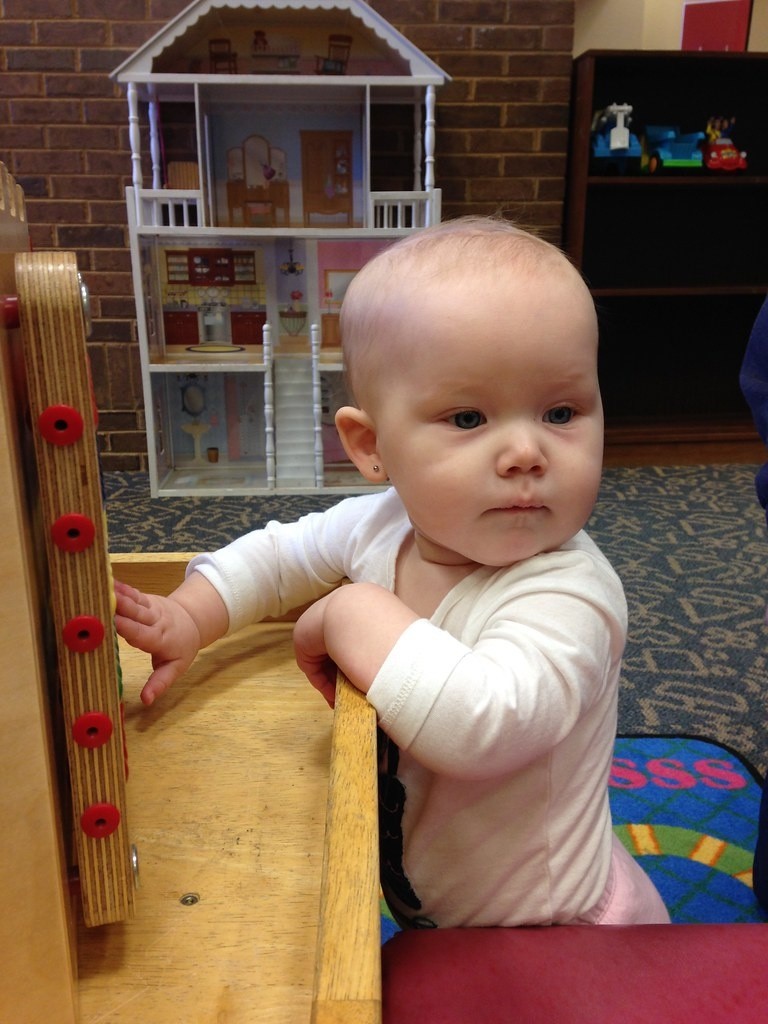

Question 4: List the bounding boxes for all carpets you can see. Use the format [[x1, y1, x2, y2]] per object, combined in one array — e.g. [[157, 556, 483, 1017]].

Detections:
[[381, 735, 767, 949], [101, 466, 767, 785]]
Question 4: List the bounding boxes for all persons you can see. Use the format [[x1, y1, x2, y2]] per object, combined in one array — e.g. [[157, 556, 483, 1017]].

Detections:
[[115, 217, 671, 929], [742, 301, 768, 915]]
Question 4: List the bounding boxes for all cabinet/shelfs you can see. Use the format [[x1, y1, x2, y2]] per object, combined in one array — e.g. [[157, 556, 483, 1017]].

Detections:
[[107, 0, 456, 498], [559, 49, 768, 469]]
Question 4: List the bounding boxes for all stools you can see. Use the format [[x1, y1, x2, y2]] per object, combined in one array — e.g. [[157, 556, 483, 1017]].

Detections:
[[381, 923, 768, 1024]]
